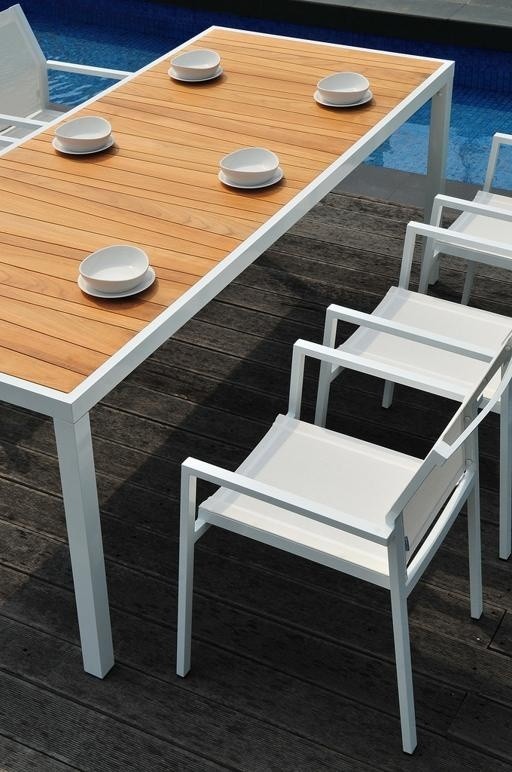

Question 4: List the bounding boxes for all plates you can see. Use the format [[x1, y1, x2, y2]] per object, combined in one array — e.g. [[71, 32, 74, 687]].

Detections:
[[168, 65, 223, 83], [218, 166, 284, 190], [52, 135, 114, 155], [313, 88, 373, 108], [77, 266, 155, 300]]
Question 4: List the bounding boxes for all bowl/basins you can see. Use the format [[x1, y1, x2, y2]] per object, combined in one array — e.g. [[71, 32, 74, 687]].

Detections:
[[220, 147, 279, 186], [54, 116, 112, 152], [170, 49, 220, 79], [317, 72, 369, 105], [78, 244, 149, 293]]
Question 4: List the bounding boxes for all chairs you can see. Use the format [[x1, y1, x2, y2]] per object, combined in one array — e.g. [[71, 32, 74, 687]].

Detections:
[[0, 4, 135, 147], [315, 220, 512, 560], [418, 132, 512, 306], [177, 331, 512, 755]]
